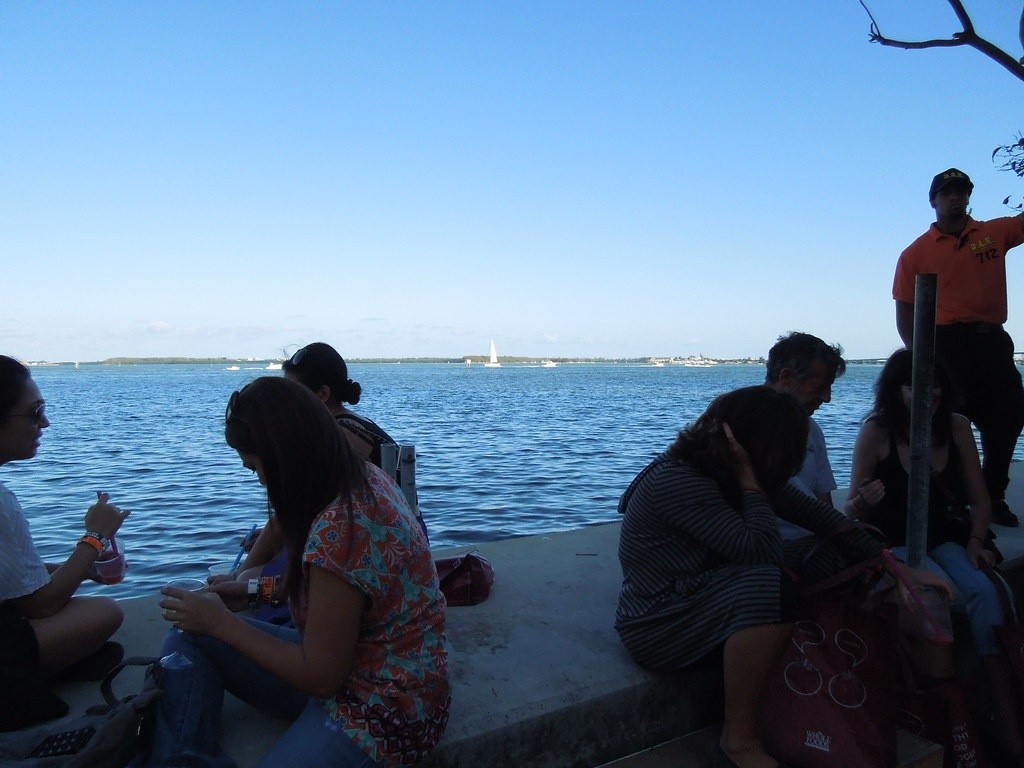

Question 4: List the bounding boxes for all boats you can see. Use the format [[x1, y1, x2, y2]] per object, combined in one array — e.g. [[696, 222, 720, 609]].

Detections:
[[266, 361, 283, 370], [225, 364, 240, 371], [541, 359, 558, 368]]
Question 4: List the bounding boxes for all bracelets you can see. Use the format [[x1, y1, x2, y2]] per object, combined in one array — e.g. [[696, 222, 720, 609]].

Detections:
[[77, 537, 102, 554], [969, 536, 984, 543], [234, 572, 236, 580], [852, 500, 862, 512], [83, 531, 110, 547]]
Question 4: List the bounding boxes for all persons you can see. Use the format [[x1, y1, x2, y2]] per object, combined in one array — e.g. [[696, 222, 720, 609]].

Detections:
[[843, 349, 1024, 768], [616, 388, 958, 768], [206, 343, 401, 586], [893, 168, 1024, 530], [152, 377, 449, 768], [763, 333, 845, 538], [0, 355, 130, 680]]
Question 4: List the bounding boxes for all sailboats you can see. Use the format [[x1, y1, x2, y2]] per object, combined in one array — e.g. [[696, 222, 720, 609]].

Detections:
[[484, 338, 501, 368]]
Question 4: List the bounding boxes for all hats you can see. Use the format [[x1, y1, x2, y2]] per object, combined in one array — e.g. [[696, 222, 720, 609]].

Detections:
[[930, 169, 974, 196]]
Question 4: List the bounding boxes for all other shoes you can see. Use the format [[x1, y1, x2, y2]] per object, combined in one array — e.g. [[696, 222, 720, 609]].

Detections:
[[47, 641, 124, 686], [712, 741, 790, 768]]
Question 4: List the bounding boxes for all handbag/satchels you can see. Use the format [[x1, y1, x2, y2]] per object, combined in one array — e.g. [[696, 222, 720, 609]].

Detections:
[[753, 519, 900, 768], [0, 658, 164, 768], [898, 688, 990, 768], [977, 559, 1024, 691], [436, 554, 489, 607]]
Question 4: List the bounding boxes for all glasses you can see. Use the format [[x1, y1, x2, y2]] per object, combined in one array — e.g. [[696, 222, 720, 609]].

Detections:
[[290, 347, 318, 380], [8, 399, 46, 424], [228, 383, 262, 444]]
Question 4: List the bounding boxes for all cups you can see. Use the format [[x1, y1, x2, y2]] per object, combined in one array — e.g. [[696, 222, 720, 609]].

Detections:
[[208, 562, 239, 576], [167, 578, 205, 632], [94, 538, 125, 585]]
